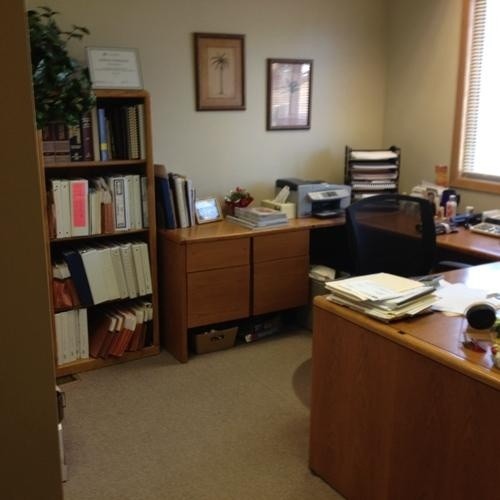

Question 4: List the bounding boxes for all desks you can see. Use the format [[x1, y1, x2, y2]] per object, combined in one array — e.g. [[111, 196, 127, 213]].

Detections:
[[157, 207, 500, 500]]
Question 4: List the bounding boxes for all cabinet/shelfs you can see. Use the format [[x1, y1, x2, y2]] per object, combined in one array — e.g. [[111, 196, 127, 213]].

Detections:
[[343, 146, 400, 210], [33, 89, 161, 379]]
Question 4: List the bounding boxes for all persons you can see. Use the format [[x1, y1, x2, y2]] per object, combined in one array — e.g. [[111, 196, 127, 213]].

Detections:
[[427, 192, 436, 216]]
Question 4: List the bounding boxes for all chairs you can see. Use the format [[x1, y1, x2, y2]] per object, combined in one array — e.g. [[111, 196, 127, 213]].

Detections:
[[346, 192, 475, 279]]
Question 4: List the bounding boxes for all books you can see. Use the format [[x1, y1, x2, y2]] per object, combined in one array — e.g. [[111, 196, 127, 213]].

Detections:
[[352, 150, 398, 199], [42, 104, 146, 163], [88, 178, 112, 236], [154, 165, 194, 231], [89, 302, 153, 360], [324, 272, 441, 324], [225, 205, 287, 229]]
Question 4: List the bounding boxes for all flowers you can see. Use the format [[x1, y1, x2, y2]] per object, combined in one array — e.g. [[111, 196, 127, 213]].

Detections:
[[221, 186, 255, 208]]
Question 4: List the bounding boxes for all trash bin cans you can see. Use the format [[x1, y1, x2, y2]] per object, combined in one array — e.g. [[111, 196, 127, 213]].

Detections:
[[295, 265, 352, 331]]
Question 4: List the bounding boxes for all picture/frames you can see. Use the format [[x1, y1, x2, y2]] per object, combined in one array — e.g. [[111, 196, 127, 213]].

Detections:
[[194, 196, 224, 224], [190, 31, 313, 132], [84, 43, 144, 92]]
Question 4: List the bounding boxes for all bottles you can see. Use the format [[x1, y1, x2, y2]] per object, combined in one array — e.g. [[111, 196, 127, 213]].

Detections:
[[446, 194, 457, 221], [435, 164, 449, 188]]
